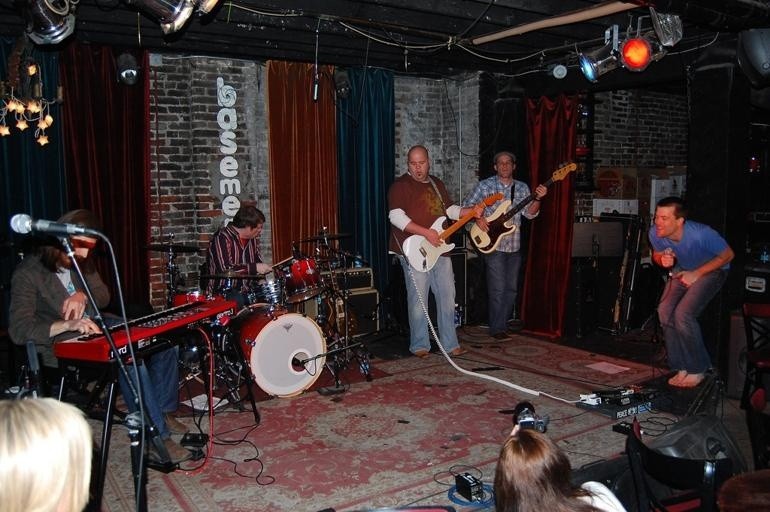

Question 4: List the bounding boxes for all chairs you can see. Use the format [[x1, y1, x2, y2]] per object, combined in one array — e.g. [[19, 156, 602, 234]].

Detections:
[[15, 251, 128, 419], [626, 416, 733, 512]]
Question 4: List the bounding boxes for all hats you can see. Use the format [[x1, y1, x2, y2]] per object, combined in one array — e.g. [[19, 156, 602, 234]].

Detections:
[[493, 151, 516, 164]]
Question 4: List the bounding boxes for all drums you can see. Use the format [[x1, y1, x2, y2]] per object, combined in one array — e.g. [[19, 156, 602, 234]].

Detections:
[[276, 257, 325, 304], [221, 303, 327, 399], [173, 286, 227, 307]]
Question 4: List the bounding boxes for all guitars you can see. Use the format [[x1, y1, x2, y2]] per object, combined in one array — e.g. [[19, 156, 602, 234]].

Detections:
[[402, 192, 506, 272], [470, 161, 577, 255], [324, 250, 357, 336]]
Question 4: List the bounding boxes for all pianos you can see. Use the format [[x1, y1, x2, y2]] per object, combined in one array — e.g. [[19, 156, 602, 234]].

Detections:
[[53, 300, 237, 363]]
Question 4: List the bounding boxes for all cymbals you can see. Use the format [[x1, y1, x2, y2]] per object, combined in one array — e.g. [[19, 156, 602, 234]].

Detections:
[[195, 271, 265, 279], [316, 257, 338, 261], [302, 233, 349, 242], [147, 244, 200, 253]]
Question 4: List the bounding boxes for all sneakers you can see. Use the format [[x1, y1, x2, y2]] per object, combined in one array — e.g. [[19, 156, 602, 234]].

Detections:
[[163, 414, 189, 435], [489, 329, 519, 342], [414, 350, 429, 358], [452, 347, 468, 355], [144, 437, 193, 464]]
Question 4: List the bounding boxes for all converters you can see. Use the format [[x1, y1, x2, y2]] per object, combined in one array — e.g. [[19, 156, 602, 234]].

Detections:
[[454, 472, 484, 503]]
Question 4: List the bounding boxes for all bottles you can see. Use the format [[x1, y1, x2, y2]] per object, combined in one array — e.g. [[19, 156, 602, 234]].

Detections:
[[455, 301, 462, 328], [354, 251, 363, 269]]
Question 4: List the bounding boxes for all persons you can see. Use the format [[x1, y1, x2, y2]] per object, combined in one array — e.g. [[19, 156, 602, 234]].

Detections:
[[492, 429, 626, 511], [201, 206, 272, 315], [7, 208, 193, 467], [463, 149, 548, 342], [647, 197, 734, 388], [0, 397, 93, 512], [387, 144, 483, 359]]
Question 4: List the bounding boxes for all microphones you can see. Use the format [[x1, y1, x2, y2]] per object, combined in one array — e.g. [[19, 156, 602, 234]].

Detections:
[[10, 213, 86, 236], [664, 247, 672, 279]]
